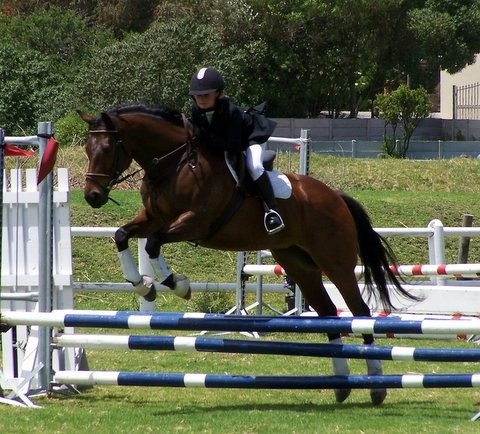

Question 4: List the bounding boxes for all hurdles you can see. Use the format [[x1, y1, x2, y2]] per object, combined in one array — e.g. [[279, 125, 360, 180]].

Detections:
[[0, 122, 480, 388]]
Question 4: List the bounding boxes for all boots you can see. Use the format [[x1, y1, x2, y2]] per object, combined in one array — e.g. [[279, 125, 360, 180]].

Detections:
[[253, 170, 281, 231]]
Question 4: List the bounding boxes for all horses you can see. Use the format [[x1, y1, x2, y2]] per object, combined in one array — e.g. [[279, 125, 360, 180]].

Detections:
[[75, 103, 424, 407]]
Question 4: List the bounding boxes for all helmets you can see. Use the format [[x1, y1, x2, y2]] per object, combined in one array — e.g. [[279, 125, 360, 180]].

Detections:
[[188, 68, 224, 98]]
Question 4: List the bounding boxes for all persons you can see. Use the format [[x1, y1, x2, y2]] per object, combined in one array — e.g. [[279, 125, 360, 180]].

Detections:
[[190, 65, 283, 229]]
